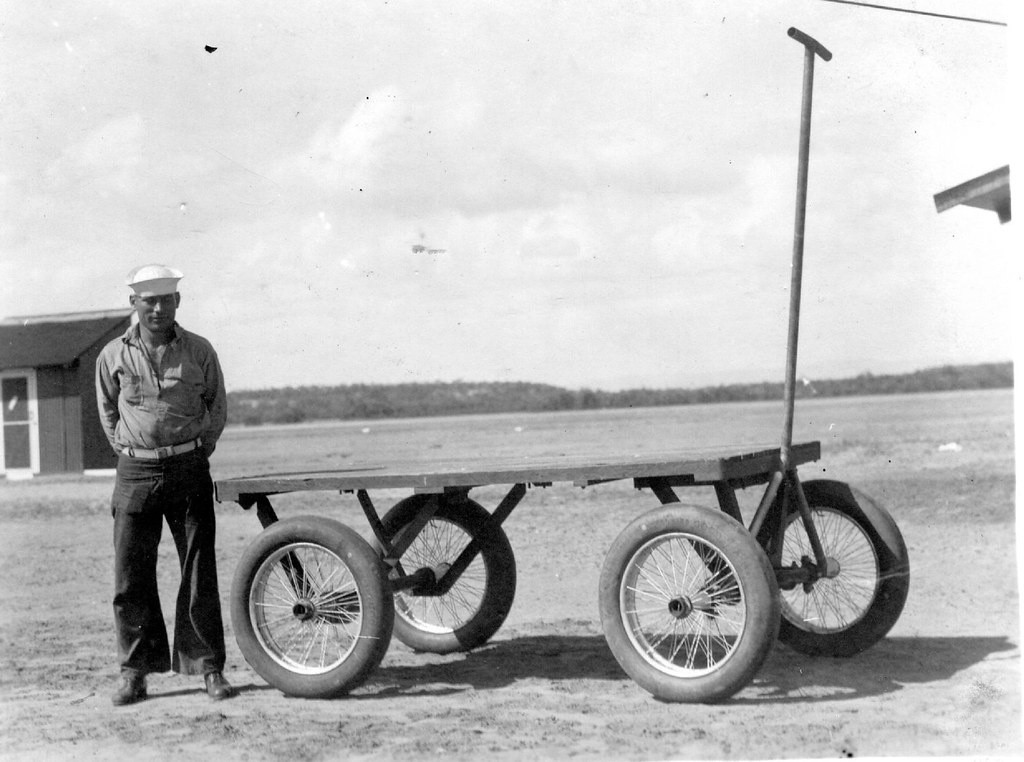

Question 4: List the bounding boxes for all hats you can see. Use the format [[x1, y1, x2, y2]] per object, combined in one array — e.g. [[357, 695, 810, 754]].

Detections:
[[126, 263, 184, 297]]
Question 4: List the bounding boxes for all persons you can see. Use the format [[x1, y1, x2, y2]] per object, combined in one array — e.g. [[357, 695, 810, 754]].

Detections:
[[95, 262, 232, 707]]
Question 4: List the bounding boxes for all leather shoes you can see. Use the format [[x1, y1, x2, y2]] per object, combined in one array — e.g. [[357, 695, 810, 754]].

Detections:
[[204, 671, 233, 699], [111, 674, 147, 706]]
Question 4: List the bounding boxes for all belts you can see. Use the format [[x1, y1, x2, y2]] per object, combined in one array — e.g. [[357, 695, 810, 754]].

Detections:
[[122, 437, 203, 459]]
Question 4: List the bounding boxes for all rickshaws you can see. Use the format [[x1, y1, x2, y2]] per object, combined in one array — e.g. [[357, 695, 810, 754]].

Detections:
[[212, 26, 910, 703]]
